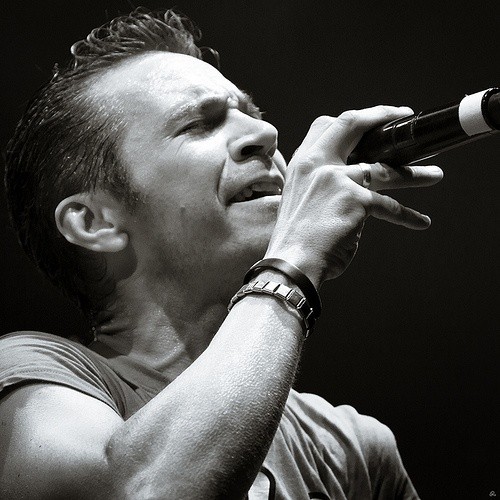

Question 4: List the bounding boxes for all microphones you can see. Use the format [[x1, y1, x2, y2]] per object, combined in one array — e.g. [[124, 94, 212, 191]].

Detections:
[[346, 87, 500, 169]]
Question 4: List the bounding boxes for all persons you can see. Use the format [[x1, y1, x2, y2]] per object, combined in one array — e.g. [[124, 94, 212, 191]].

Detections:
[[0, 8, 444, 500]]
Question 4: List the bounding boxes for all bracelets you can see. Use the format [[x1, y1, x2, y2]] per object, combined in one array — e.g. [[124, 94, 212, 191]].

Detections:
[[227, 258, 323, 338]]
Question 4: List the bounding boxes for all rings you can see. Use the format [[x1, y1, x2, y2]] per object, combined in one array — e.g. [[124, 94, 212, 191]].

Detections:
[[359, 163, 371, 189]]
